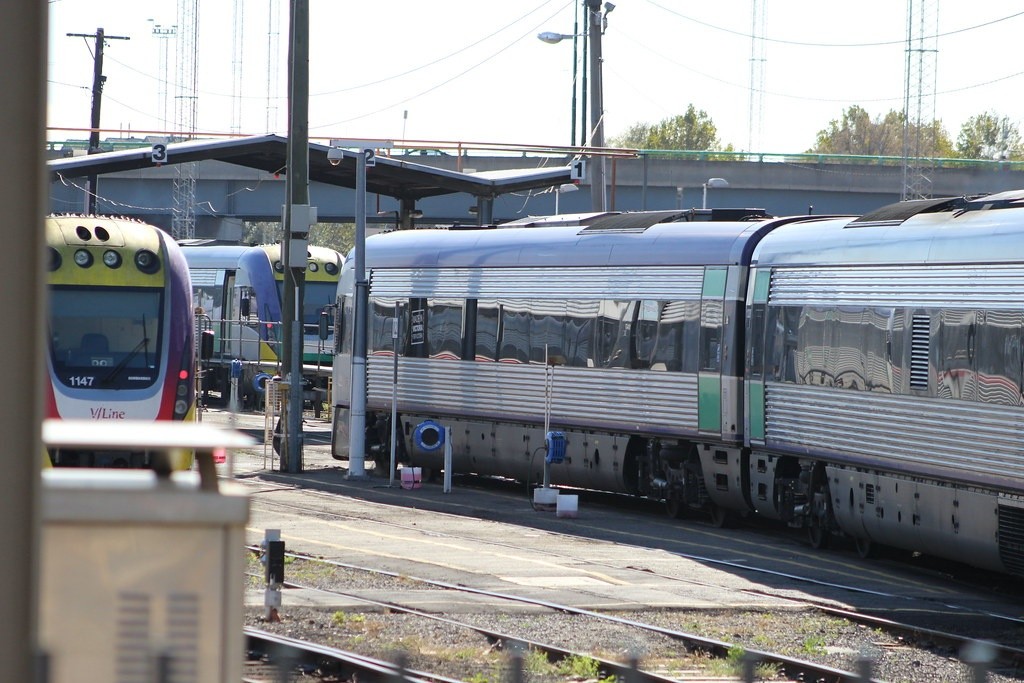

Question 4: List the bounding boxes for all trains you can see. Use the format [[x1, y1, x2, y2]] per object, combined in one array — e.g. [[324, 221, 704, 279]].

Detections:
[[176, 237, 345, 409], [319, 188, 1024, 582], [45, 212, 215, 474]]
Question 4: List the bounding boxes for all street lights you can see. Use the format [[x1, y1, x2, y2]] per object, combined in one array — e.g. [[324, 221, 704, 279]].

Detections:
[[537, 0, 608, 215], [326, 140, 393, 483], [700, 177, 731, 210], [555, 183, 578, 216]]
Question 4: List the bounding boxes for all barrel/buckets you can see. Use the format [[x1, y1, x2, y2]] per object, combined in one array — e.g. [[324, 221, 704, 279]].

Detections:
[[534, 489, 559, 512], [557, 494, 578, 519], [400, 467, 421, 490]]
